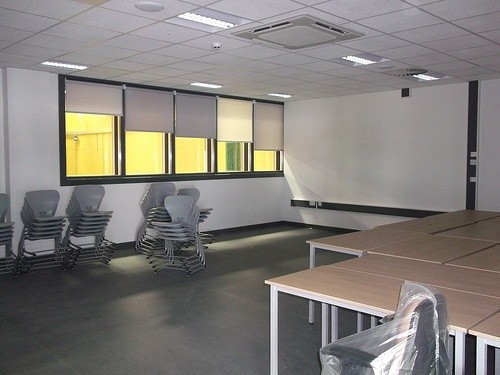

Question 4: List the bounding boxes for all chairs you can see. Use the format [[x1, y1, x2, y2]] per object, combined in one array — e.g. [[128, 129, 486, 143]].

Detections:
[[0, 182, 215, 280]]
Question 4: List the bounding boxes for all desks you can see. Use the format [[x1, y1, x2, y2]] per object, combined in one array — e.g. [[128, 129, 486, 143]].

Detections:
[[264, 208, 500, 375]]
